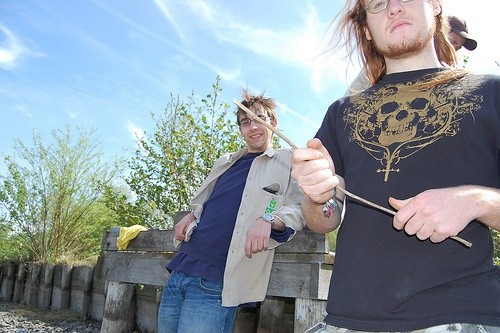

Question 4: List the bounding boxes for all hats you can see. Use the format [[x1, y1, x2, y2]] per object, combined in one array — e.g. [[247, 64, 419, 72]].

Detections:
[[447, 15, 477, 52]]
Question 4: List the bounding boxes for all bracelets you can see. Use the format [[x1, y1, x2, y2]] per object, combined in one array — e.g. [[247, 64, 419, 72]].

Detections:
[[315, 187, 338, 217]]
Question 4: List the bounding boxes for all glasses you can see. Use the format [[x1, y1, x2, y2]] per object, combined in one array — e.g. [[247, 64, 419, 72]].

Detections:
[[360, 0, 414, 14], [238, 116, 272, 129]]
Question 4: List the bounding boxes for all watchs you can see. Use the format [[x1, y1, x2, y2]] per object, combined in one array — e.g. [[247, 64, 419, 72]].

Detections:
[[258, 214, 275, 228]]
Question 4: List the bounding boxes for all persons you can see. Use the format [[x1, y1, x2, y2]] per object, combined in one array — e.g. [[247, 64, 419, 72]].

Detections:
[[290, 0, 500, 333], [344, 16, 477, 98], [157, 92, 306, 333]]
